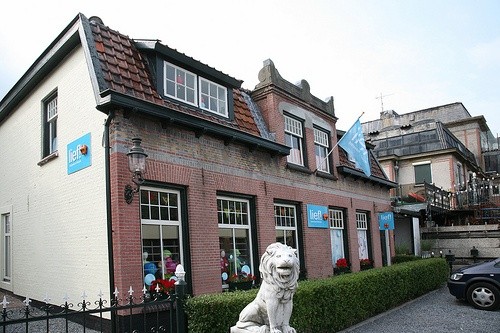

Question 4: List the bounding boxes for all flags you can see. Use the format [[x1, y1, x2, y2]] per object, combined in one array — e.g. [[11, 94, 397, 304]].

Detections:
[[337, 119, 372, 177]]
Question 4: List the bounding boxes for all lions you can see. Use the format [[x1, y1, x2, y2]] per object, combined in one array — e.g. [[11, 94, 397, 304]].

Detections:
[[236, 240, 300, 333]]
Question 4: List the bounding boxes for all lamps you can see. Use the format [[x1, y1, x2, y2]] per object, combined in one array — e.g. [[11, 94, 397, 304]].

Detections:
[[124, 138, 148, 205], [391, 197, 401, 213]]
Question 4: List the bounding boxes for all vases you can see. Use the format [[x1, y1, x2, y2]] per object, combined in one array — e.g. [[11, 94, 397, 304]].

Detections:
[[362, 265, 374, 270], [229, 281, 253, 291], [334, 267, 348, 274]]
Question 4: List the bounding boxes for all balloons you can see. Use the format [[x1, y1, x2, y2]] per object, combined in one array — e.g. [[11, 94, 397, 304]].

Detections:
[[144, 257, 178, 286]]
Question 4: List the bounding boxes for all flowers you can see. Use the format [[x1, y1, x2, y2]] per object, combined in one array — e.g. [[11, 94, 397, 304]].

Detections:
[[336, 258, 349, 269], [361, 259, 372, 265], [226, 271, 256, 284], [149, 274, 176, 294]]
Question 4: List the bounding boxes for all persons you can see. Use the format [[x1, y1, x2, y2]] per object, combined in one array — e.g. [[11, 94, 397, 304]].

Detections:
[[220, 249, 229, 273]]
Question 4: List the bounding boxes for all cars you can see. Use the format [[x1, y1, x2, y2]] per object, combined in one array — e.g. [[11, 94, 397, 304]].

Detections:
[[447, 257, 500, 312]]
